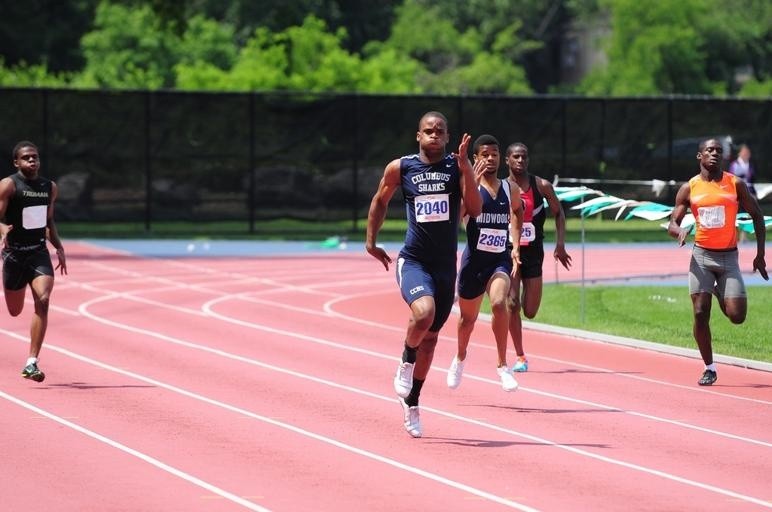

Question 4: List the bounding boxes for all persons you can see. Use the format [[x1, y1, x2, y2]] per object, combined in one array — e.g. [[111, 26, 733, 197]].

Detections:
[[0, 139, 68, 382], [503, 142, 573, 373], [362, 110, 486, 439], [730, 143, 760, 208], [668, 137, 769, 387], [446, 133, 526, 392]]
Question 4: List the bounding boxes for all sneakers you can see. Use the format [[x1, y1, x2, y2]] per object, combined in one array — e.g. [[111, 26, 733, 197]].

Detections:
[[447, 351, 467, 389], [22, 363, 44, 381], [394, 358, 416, 398], [399, 397, 422, 438], [497, 365, 518, 392], [513, 356, 528, 372], [698, 369, 717, 386]]
